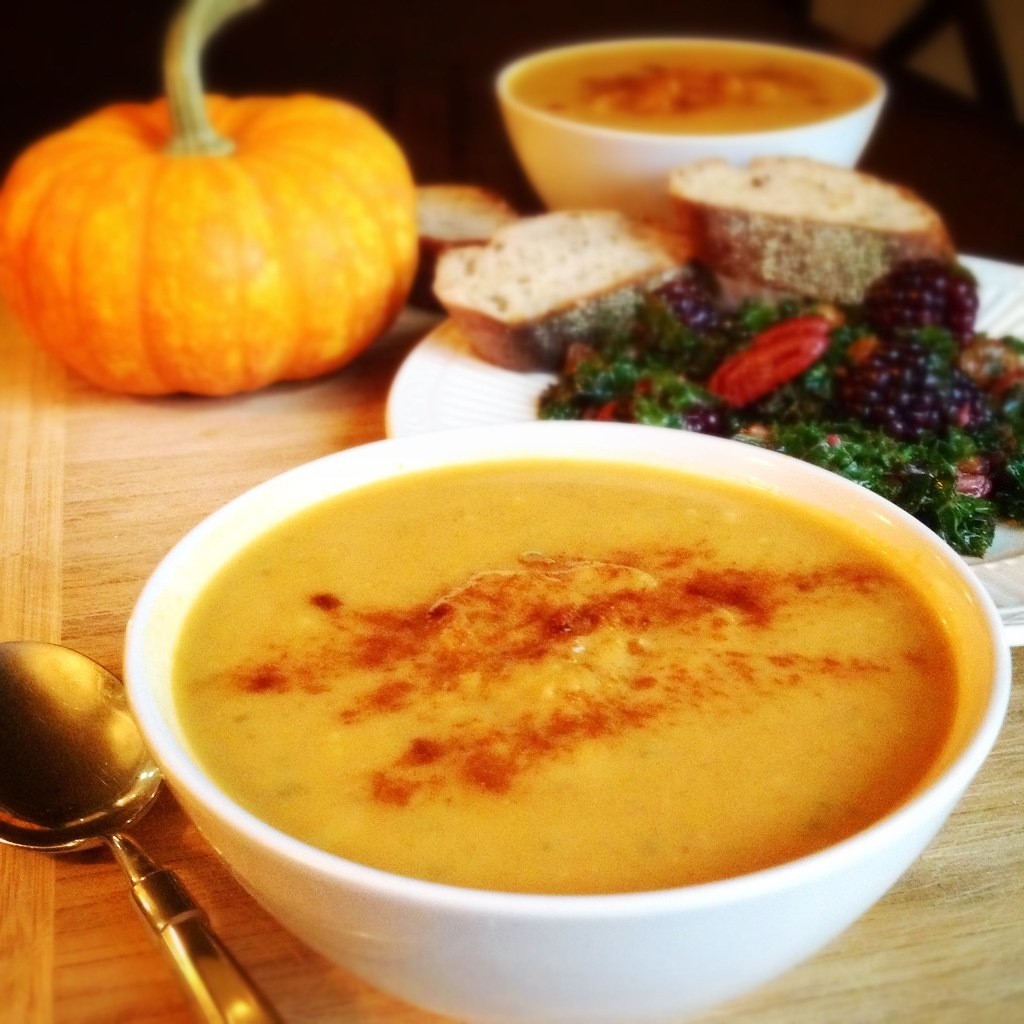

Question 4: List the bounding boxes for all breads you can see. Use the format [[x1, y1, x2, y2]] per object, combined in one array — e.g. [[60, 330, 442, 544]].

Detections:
[[667, 155, 956, 303], [429, 208, 697, 373], [407, 182, 519, 306]]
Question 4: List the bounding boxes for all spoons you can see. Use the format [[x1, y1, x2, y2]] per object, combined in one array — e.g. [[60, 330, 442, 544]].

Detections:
[[0, 642, 283, 1024]]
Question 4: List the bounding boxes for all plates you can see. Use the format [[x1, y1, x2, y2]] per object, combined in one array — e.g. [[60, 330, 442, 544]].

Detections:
[[385, 253, 1024, 646]]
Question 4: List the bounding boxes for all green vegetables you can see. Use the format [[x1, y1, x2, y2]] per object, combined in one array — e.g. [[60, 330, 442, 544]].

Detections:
[[534, 293, 1024, 557]]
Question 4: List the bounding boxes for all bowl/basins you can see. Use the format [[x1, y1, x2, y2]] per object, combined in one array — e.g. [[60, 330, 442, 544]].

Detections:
[[497, 36, 887, 210], [121, 421, 1011, 1024]]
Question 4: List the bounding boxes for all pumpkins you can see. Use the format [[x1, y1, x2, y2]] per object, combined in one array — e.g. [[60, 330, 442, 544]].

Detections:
[[0, 0, 423, 400]]
